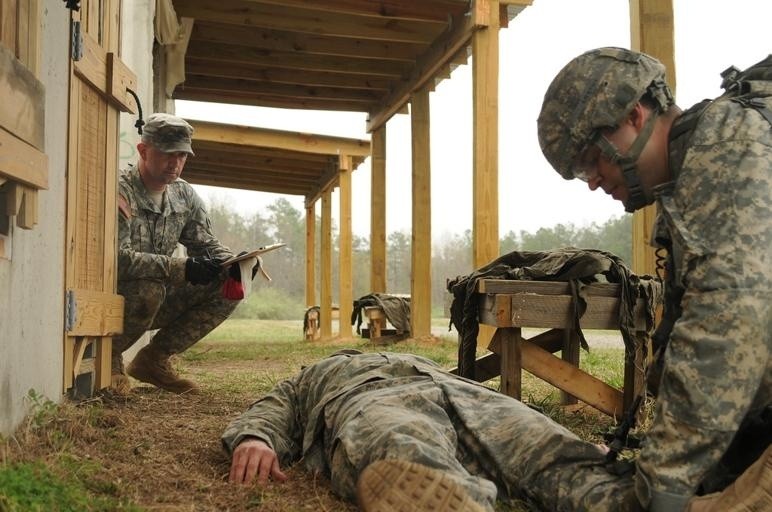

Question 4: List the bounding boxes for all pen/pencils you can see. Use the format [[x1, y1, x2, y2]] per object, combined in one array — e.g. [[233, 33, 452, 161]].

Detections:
[[204, 248, 212, 259]]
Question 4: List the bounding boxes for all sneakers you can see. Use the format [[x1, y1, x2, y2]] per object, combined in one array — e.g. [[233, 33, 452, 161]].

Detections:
[[127, 344, 201, 393], [358, 459, 486, 512]]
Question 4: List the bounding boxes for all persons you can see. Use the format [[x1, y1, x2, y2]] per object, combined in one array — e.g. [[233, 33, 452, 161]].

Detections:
[[221, 349, 772, 512], [111, 114, 262, 396], [537, 47, 771, 512]]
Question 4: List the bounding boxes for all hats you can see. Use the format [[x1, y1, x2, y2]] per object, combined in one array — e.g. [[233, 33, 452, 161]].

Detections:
[[143, 113, 194, 155]]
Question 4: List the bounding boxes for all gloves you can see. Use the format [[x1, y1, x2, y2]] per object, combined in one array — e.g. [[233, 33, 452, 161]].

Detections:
[[186, 256, 224, 285], [229, 251, 257, 281]]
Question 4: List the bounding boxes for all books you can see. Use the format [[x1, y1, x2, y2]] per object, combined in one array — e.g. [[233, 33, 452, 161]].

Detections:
[[221, 243, 286, 267]]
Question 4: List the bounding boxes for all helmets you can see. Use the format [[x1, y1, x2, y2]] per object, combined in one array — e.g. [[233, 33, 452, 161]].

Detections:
[[538, 47, 666, 180]]
